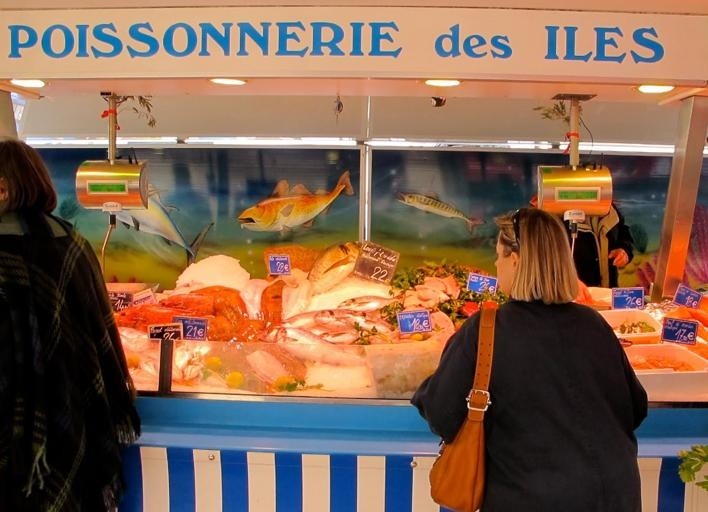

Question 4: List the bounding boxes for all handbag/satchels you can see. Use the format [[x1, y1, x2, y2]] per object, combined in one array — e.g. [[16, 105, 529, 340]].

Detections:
[[428, 389, 490, 511]]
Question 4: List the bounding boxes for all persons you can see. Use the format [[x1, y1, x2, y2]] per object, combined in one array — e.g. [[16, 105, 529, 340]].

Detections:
[[405, 204, 651, 512], [528, 192, 634, 290], [0, 137, 143, 512], [143, 149, 538, 247]]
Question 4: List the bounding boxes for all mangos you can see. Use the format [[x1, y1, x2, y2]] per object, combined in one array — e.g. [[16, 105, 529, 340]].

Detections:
[[225, 372, 244, 389], [206, 357, 222, 370]]
